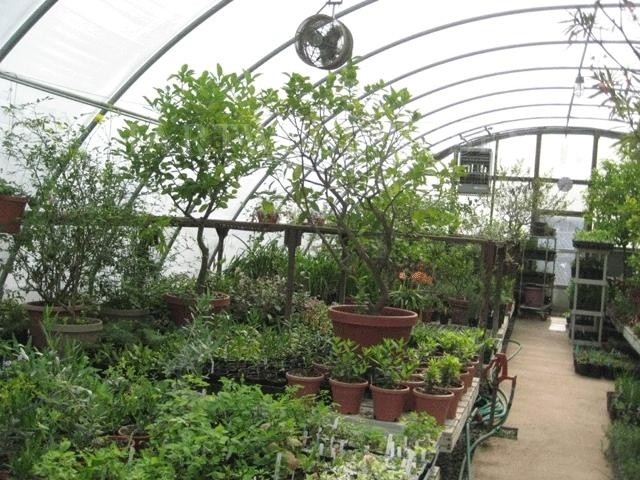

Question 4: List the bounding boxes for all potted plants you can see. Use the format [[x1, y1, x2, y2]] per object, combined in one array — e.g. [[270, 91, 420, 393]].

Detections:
[[560, 0, 640, 479], [525, 263, 556, 282], [0, 53, 517, 479], [524, 240, 557, 261]]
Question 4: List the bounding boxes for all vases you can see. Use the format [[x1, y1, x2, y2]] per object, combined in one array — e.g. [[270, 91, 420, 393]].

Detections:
[[524, 286, 545, 307], [533, 222, 548, 235]]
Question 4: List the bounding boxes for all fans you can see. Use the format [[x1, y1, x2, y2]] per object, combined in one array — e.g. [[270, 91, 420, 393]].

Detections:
[[295, 0, 355, 71]]
